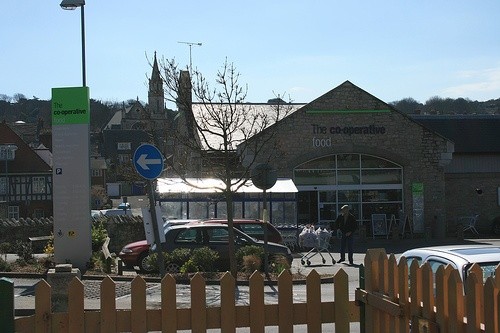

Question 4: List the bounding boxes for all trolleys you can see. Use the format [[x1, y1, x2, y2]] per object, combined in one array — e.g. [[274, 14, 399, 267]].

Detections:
[[298, 230, 336, 266]]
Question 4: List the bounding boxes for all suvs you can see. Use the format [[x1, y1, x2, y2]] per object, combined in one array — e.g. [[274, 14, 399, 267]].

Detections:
[[395, 244, 500, 308], [119, 218, 293, 274]]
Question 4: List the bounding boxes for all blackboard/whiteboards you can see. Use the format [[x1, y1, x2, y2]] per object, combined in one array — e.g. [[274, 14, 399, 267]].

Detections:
[[399, 215, 412, 238], [372, 214, 387, 235]]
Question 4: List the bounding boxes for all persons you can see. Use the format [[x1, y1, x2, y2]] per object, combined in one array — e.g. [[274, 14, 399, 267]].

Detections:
[[331, 205, 357, 266]]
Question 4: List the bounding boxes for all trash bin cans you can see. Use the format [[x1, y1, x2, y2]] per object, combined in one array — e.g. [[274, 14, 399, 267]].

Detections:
[[47, 264, 81, 312]]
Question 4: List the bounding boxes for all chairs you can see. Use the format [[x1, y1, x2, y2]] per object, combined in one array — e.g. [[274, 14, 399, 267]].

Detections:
[[456, 215, 480, 234]]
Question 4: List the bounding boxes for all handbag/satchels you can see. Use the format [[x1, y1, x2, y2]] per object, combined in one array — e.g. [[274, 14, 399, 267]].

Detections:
[[299, 225, 328, 239]]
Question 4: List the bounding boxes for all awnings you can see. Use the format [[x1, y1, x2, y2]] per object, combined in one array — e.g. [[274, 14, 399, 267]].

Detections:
[[156, 177, 298, 197]]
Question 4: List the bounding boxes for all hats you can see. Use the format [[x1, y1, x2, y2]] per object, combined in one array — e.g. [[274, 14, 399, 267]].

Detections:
[[341, 205, 348, 209]]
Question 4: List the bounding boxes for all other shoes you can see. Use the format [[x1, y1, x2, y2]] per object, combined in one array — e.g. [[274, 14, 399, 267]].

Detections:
[[337, 259, 345, 263], [348, 263, 353, 266]]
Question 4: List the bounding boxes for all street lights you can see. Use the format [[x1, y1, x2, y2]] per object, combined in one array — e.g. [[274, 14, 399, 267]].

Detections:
[[60, 0, 87, 87]]
